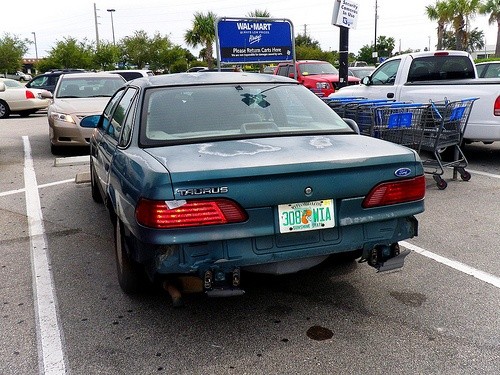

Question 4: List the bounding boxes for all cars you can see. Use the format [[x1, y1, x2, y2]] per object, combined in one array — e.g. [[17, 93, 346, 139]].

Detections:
[[0, 78, 54, 119], [337, 61, 393, 85], [40, 73, 129, 155], [80, 72, 427, 295], [464, 61, 500, 78]]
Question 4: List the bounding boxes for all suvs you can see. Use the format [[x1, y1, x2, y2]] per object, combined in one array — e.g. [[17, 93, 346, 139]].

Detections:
[[0, 67, 243, 94]]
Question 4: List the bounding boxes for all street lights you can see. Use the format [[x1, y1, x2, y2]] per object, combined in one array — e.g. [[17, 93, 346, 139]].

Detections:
[[107, 9, 115, 48], [32, 32, 38, 60]]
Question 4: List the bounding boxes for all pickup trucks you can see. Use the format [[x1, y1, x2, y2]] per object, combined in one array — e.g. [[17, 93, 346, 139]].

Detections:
[[328, 50, 500, 161], [273, 60, 362, 99]]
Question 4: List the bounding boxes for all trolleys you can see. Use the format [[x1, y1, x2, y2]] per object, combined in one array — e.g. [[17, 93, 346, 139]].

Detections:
[[321, 97, 481, 190]]
